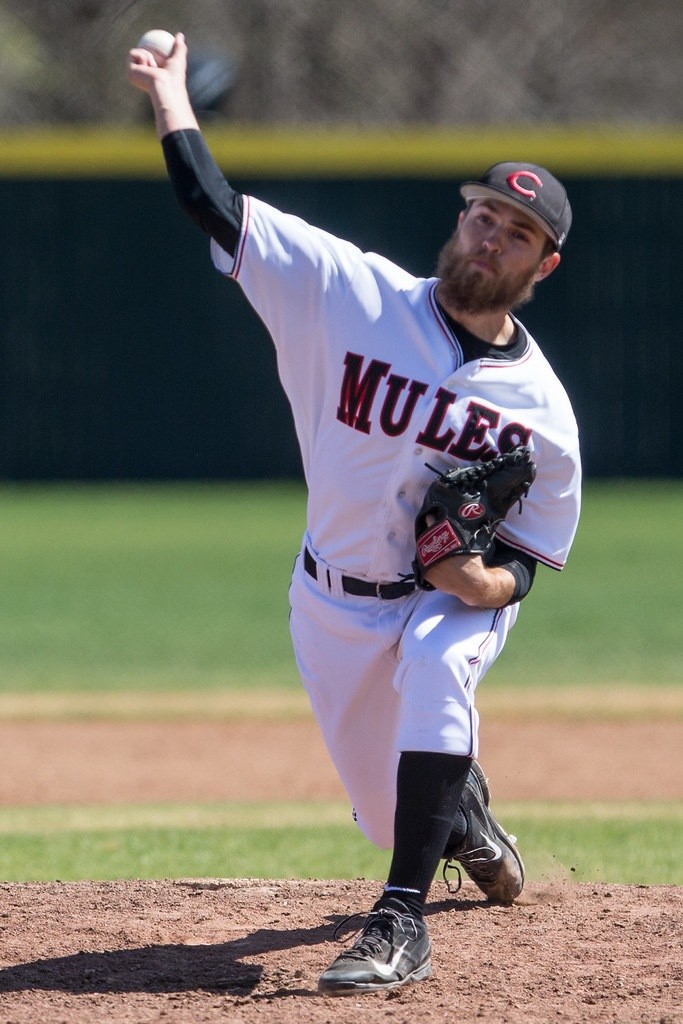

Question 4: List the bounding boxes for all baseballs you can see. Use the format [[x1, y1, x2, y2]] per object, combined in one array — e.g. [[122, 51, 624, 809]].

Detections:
[[137, 29, 176, 66]]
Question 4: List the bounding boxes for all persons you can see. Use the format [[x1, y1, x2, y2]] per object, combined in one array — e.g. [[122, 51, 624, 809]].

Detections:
[[127, 32, 583, 997]]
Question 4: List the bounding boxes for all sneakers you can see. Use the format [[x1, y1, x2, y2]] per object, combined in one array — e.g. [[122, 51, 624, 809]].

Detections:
[[317, 897, 432, 995], [443, 760, 525, 900]]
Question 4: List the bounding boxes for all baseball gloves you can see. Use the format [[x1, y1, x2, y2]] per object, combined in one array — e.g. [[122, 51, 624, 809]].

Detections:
[[411, 445, 538, 593]]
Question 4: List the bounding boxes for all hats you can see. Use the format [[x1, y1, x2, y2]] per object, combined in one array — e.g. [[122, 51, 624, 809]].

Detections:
[[460, 162, 573, 252]]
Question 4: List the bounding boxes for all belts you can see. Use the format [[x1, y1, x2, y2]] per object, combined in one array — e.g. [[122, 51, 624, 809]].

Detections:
[[304, 545, 417, 599]]
